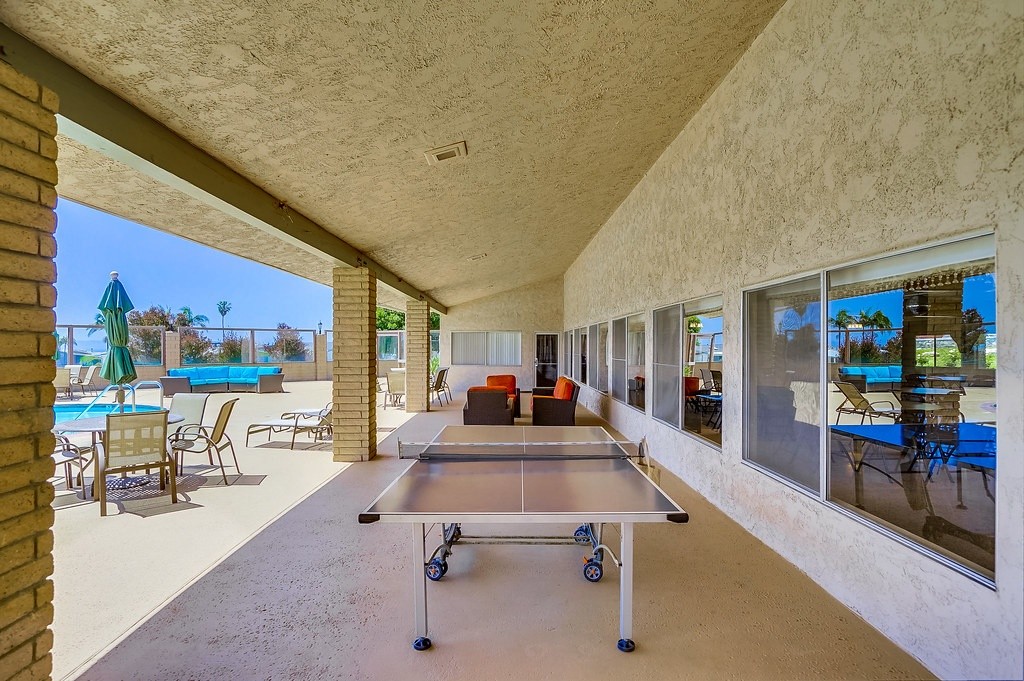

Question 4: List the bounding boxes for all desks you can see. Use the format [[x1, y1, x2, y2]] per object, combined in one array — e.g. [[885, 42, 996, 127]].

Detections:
[[54, 414, 185, 496], [358, 426, 689, 651], [829, 423, 997, 518]]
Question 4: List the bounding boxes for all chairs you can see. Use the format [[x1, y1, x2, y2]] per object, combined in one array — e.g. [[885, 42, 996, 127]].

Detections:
[[168, 397, 240, 485], [52, 430, 94, 500], [246, 402, 333, 449], [64, 365, 84, 394], [52, 368, 73, 401], [699, 369, 722, 395], [384, 367, 452, 410], [73, 366, 98, 395], [832, 380, 901, 425], [95, 411, 177, 516], [168, 392, 213, 476]]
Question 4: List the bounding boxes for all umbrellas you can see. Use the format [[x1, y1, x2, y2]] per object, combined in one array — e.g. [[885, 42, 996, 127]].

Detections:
[[97, 271, 137, 479]]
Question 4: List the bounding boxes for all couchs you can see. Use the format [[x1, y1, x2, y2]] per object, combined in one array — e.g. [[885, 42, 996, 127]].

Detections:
[[463, 386, 514, 425], [487, 375, 520, 418], [160, 365, 285, 397], [531, 376, 580, 426], [838, 365, 902, 393]]
